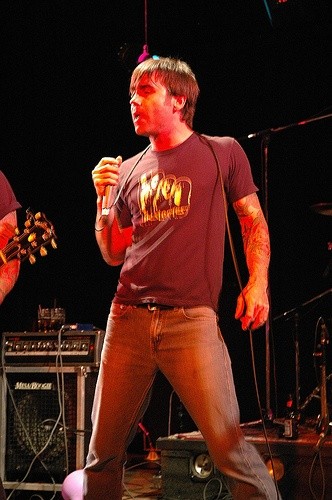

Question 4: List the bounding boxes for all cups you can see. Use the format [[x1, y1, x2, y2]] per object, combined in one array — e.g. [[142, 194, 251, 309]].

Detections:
[[38, 308, 51, 332], [50, 308, 66, 332]]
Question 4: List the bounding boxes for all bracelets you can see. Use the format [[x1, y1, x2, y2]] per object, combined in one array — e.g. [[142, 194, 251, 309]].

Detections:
[[97, 196, 104, 202]]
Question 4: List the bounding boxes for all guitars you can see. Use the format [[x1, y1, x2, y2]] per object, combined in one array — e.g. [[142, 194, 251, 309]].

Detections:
[[0, 207, 58, 272]]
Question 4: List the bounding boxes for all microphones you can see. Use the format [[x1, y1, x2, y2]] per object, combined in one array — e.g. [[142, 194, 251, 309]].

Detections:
[[102, 163, 118, 225]]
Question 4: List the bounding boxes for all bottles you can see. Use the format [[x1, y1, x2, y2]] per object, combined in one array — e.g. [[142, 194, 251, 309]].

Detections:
[[283, 392, 299, 439]]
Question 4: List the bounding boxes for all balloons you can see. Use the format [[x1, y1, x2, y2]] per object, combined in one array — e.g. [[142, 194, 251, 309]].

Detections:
[[62, 469, 84, 500]]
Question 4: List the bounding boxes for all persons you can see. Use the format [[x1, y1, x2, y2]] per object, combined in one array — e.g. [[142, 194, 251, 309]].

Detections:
[[0, 172, 22, 304], [82, 59, 282, 500]]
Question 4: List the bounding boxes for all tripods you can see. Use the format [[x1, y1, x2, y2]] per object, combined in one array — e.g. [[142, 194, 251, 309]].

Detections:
[[238, 320, 286, 428]]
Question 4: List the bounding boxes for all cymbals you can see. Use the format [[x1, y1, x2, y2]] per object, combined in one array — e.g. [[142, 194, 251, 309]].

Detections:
[[310, 202, 332, 216]]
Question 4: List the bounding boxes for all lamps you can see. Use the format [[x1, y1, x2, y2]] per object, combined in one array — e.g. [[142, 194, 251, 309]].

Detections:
[[138, 0, 150, 63]]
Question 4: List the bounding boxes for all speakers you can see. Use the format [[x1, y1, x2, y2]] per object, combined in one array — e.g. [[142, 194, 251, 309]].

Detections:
[[1, 367, 98, 491]]
